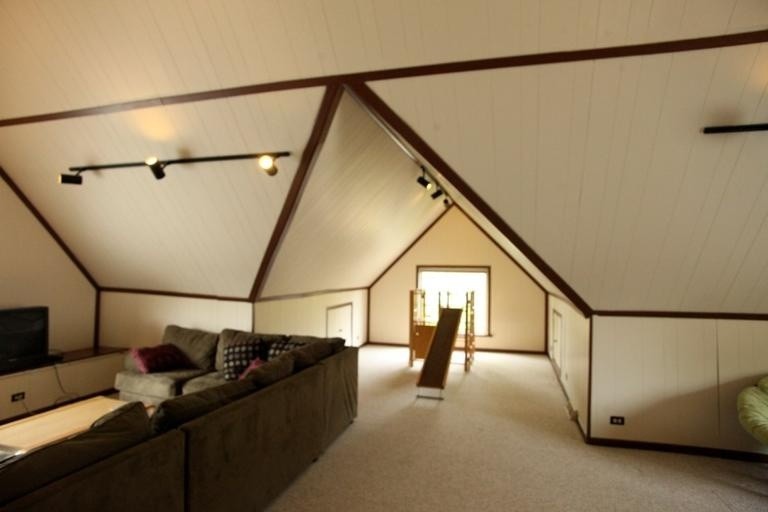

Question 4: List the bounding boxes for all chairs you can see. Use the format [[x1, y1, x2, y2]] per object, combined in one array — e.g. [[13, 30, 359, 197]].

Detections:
[[736, 375, 768, 446]]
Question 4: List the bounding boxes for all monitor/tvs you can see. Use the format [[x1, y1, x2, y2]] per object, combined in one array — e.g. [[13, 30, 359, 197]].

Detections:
[[0, 306, 51, 375]]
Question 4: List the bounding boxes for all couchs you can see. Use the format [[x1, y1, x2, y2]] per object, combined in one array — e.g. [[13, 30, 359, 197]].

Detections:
[[0, 347, 357, 512]]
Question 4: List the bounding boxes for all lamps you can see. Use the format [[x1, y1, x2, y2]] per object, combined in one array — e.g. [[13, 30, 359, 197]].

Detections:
[[417, 166, 449, 208], [58, 153, 289, 184]]
